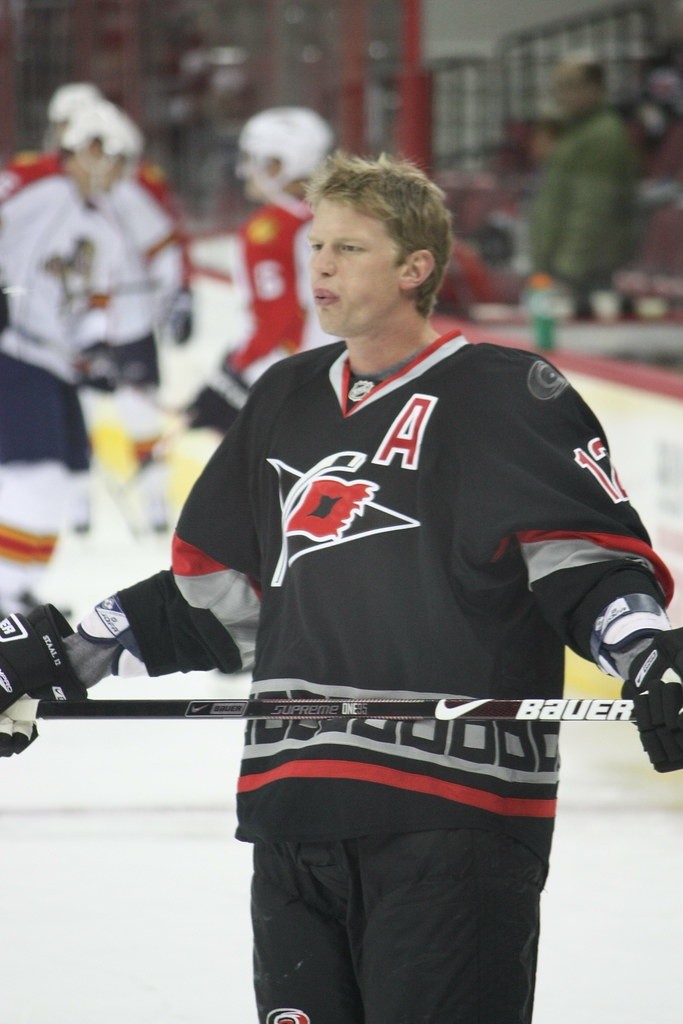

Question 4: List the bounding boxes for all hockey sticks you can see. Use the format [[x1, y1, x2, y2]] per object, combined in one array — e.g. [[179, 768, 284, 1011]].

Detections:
[[2, 690, 683, 722]]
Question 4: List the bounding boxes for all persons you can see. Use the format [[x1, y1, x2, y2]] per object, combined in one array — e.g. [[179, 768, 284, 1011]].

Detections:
[[1, 80, 192, 619], [1, 160, 682, 1024], [527, 49, 639, 320], [182, 107, 338, 672]]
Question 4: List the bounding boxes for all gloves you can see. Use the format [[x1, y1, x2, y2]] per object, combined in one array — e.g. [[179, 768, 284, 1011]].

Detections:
[[172, 290, 192, 343], [79, 342, 117, 393], [621, 627, 683, 773], [187, 360, 249, 434], [0, 604, 88, 757]]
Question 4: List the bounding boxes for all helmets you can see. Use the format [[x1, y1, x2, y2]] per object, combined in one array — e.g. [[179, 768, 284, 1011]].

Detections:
[[61, 97, 143, 162], [46, 81, 102, 123], [235, 105, 333, 221]]
